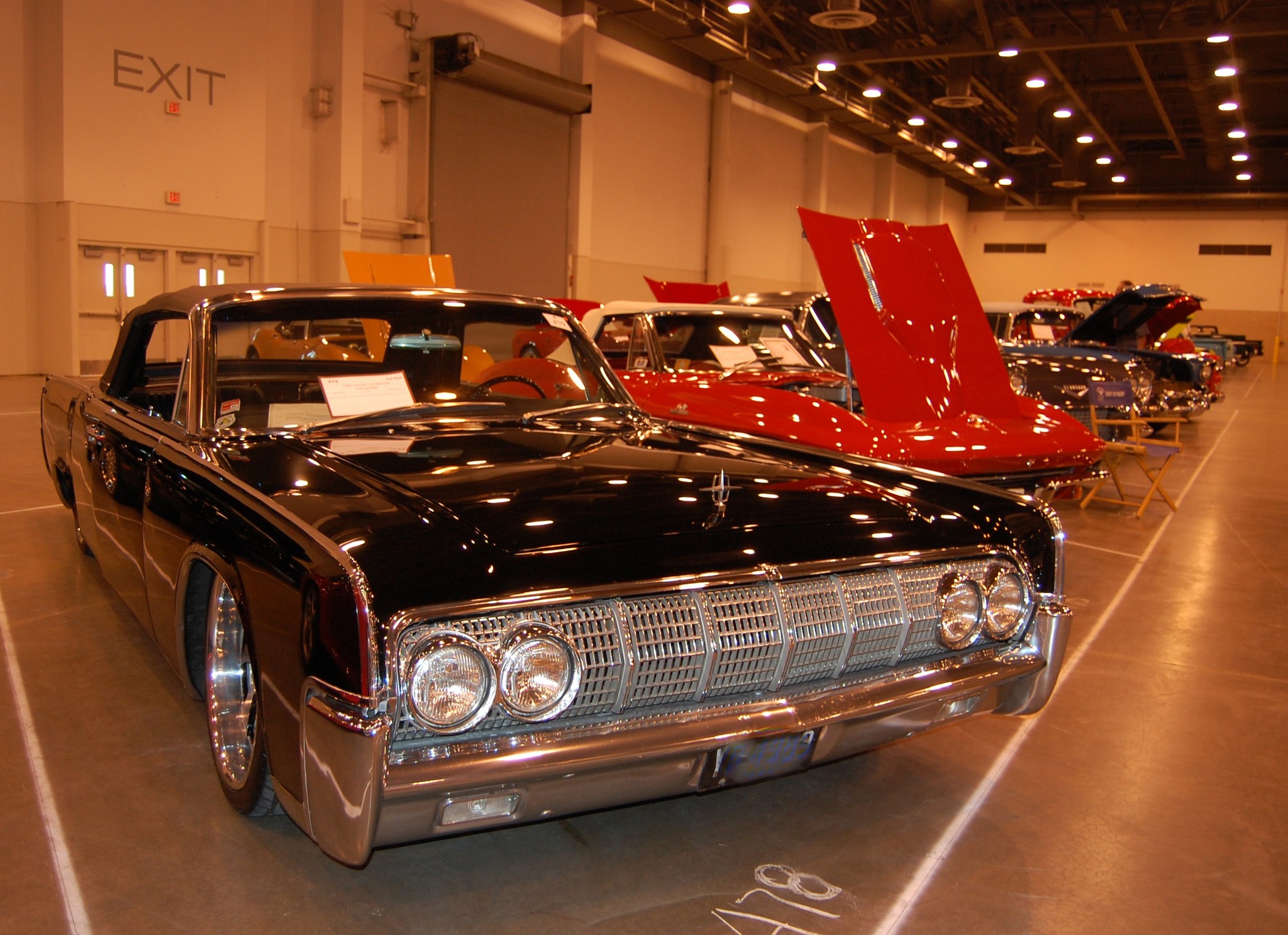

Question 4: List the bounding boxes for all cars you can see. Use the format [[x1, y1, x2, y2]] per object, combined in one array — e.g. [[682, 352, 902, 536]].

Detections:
[[474, 208, 1262, 501], [243, 253, 494, 388], [38, 284, 1074, 870]]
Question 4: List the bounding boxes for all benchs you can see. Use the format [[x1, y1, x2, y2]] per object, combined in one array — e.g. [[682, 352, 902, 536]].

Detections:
[[137, 375, 324, 414]]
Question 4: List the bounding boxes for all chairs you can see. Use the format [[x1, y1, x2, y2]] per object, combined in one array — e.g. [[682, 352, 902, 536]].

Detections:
[[1078, 378, 1187, 520]]
